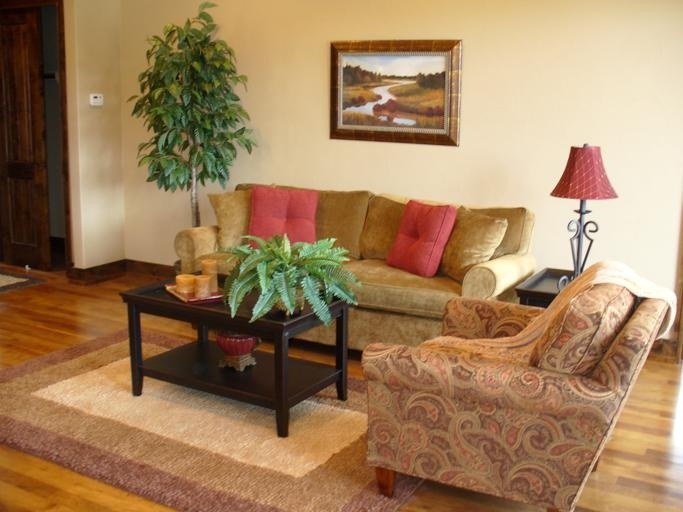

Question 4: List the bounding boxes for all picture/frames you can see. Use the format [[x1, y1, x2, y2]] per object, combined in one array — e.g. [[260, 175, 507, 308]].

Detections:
[[329, 39, 462, 147]]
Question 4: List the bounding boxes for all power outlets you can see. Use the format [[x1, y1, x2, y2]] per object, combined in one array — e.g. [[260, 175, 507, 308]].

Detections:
[[89, 94, 104, 106]]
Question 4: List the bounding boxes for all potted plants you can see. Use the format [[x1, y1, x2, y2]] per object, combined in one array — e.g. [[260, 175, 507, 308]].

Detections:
[[210, 231, 364, 330], [123, 0, 259, 275]]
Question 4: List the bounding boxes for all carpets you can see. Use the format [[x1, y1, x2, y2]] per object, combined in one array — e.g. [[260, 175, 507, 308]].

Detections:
[[1, 323, 425, 512]]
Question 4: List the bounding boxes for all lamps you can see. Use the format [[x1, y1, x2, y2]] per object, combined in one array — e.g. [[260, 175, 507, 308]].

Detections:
[[549, 143, 619, 290]]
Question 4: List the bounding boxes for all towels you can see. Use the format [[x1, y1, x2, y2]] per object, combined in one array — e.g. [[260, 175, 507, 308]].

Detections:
[[421, 254, 679, 348]]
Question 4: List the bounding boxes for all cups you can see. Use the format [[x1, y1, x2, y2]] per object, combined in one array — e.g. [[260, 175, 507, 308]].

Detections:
[[201, 259, 218, 293], [175, 274, 194, 293], [194, 275, 211, 299]]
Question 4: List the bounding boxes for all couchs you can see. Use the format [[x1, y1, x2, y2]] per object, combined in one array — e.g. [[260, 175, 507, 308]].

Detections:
[[361, 256, 674, 512], [172, 182, 538, 352]]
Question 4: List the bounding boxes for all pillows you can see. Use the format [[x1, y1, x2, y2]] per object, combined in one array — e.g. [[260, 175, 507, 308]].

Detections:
[[202, 189, 252, 250], [527, 257, 638, 377], [384, 200, 459, 279], [441, 205, 509, 284], [248, 185, 320, 250]]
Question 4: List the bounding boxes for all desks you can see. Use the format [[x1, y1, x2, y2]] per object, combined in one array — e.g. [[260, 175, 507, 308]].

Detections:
[[514, 266, 585, 307]]
